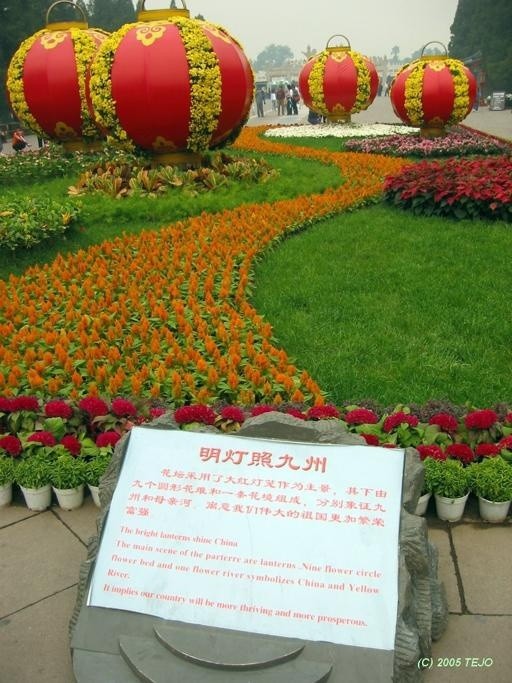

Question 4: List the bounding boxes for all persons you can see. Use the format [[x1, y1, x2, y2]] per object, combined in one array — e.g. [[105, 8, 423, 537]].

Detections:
[[12, 128, 33, 151], [376, 74, 396, 96], [255, 79, 303, 118], [308, 108, 323, 124]]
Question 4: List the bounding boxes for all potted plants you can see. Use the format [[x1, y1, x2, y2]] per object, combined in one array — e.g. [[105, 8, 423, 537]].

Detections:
[[468, 455, 512, 523], [51, 455, 85, 511], [414, 456, 433, 516], [0, 453, 15, 506], [433, 459, 470, 522], [15, 455, 52, 511], [85, 454, 111, 508]]
